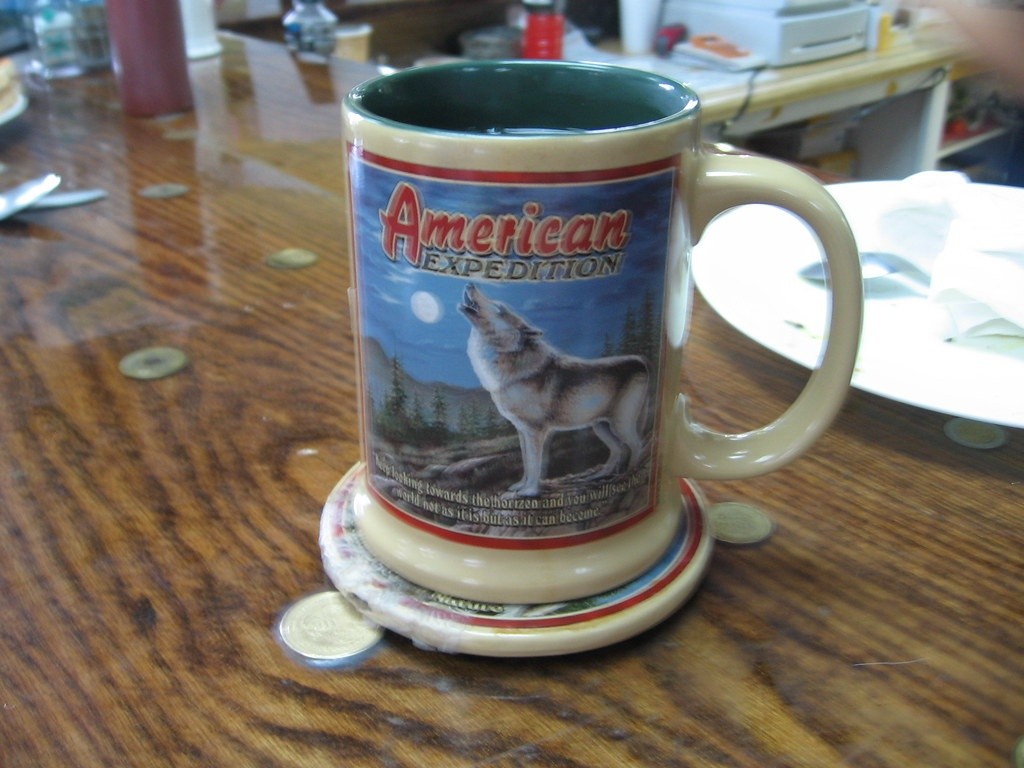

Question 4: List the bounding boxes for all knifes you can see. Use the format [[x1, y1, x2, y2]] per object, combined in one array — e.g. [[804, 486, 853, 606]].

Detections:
[[21, 188, 107, 209]]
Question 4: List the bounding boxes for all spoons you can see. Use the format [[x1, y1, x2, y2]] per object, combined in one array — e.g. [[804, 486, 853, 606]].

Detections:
[[0, 174, 61, 223]]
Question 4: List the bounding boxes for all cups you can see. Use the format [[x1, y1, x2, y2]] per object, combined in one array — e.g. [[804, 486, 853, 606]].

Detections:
[[105, 0, 196, 116], [620, 0, 663, 53], [337, 57, 864, 605], [331, 22, 371, 58]]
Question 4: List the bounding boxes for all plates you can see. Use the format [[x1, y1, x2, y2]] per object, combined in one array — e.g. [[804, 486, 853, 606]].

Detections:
[[319, 461, 718, 655], [694, 180, 1022, 432], [1, 94, 28, 129]]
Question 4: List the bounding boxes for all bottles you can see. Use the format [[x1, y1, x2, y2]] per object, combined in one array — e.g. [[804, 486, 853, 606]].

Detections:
[[285, 1, 339, 59], [521, 2, 564, 65], [18, 1, 87, 78]]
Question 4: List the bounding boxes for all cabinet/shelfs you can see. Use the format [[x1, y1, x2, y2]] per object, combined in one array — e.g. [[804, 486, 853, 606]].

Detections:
[[589, 0, 1023, 187]]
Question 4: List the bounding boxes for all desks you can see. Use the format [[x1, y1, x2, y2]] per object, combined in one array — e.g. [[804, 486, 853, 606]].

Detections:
[[1, 0, 1024, 766]]
